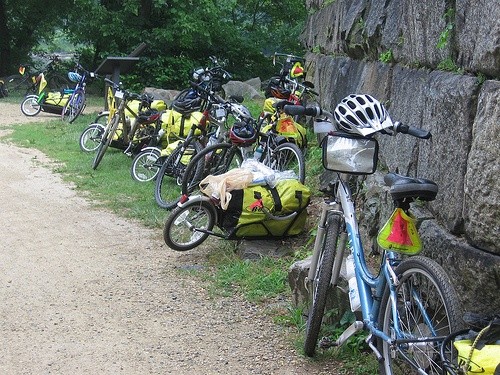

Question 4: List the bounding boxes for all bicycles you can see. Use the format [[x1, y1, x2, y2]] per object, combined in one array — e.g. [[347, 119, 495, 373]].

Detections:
[[0, 54, 102, 123], [130, 51, 320, 250], [284, 104, 470, 375], [79, 56, 168, 170]]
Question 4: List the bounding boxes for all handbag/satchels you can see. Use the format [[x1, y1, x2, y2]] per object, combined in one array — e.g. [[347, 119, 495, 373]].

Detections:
[[39, 90, 75, 116], [210, 170, 312, 241], [102, 88, 221, 167]]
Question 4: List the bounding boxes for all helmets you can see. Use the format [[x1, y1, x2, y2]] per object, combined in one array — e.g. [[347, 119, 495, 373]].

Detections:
[[229, 122, 259, 148], [332, 92, 395, 138], [173, 87, 205, 116], [137, 106, 161, 124], [269, 78, 291, 99]]
